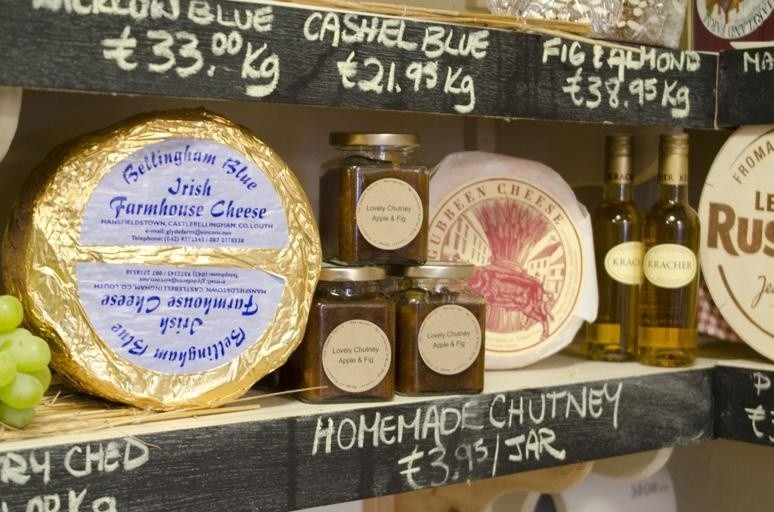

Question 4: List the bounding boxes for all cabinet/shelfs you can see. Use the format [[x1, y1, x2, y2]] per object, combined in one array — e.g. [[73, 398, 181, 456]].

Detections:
[[2, 0, 774, 511]]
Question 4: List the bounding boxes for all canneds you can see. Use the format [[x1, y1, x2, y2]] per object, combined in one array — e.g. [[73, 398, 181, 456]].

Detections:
[[385, 261, 486, 394], [318, 133, 430, 267], [299, 267, 396, 404]]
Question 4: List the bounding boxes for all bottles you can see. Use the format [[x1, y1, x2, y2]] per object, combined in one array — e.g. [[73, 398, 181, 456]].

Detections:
[[639, 132, 700, 370], [582, 131, 639, 363]]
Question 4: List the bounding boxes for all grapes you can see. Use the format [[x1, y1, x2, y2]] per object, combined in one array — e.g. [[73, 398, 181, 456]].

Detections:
[[0, 295, 51, 428]]
